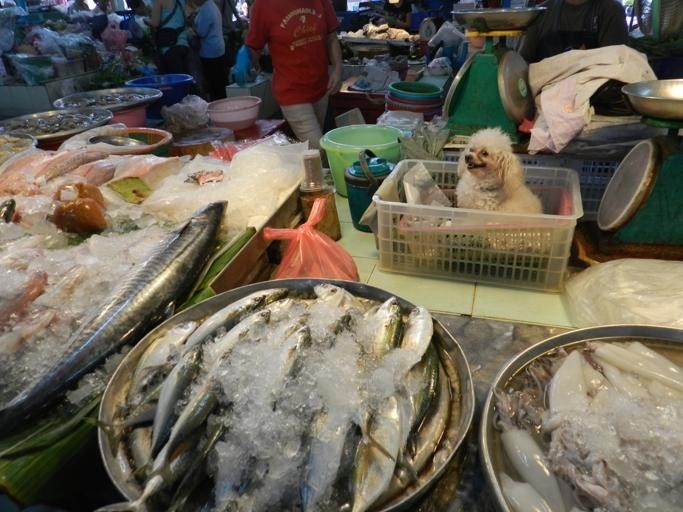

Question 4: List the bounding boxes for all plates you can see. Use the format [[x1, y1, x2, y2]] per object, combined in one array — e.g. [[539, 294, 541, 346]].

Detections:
[[95, 279, 476, 512], [480, 323, 683, 512]]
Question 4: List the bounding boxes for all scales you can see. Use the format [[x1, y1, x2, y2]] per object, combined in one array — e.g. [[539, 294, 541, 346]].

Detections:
[[419, 16, 442, 44], [584, 79, 682, 245], [443, 6, 549, 145]]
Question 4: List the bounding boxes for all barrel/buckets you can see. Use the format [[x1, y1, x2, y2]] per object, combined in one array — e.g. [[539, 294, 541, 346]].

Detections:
[[319, 123, 407, 198], [125, 73, 195, 119], [384, 82, 442, 123]]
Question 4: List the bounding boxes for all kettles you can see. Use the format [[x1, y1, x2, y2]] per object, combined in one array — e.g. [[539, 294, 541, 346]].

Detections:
[[343, 148, 397, 233]]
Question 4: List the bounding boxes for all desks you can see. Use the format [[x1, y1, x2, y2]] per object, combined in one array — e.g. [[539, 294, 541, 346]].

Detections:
[[323, 168, 582, 512], [235, 119, 287, 141]]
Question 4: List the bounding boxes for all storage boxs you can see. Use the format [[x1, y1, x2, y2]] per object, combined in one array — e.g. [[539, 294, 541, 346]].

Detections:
[[444, 150, 619, 222], [373, 159, 584, 294]]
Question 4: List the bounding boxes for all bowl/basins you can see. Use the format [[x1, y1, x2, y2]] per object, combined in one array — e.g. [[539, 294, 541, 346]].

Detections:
[[0, 106, 113, 144], [52, 88, 163, 113], [205, 96, 262, 132], [84, 127, 173, 161], [386, 80, 443, 126]]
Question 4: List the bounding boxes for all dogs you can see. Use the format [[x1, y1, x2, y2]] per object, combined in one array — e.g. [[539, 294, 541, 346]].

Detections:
[[455, 127, 544, 259]]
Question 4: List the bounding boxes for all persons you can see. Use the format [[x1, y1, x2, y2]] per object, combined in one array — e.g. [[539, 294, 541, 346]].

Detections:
[[129, 0, 151, 39], [100, 0, 131, 30], [191, 0, 227, 102], [245, 0, 343, 169], [380, 0, 413, 27], [151, 1, 201, 74]]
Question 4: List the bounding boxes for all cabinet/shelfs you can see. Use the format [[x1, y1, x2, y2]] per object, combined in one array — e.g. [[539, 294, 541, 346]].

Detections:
[[322, 36, 411, 134], [0, 68, 99, 116]]
